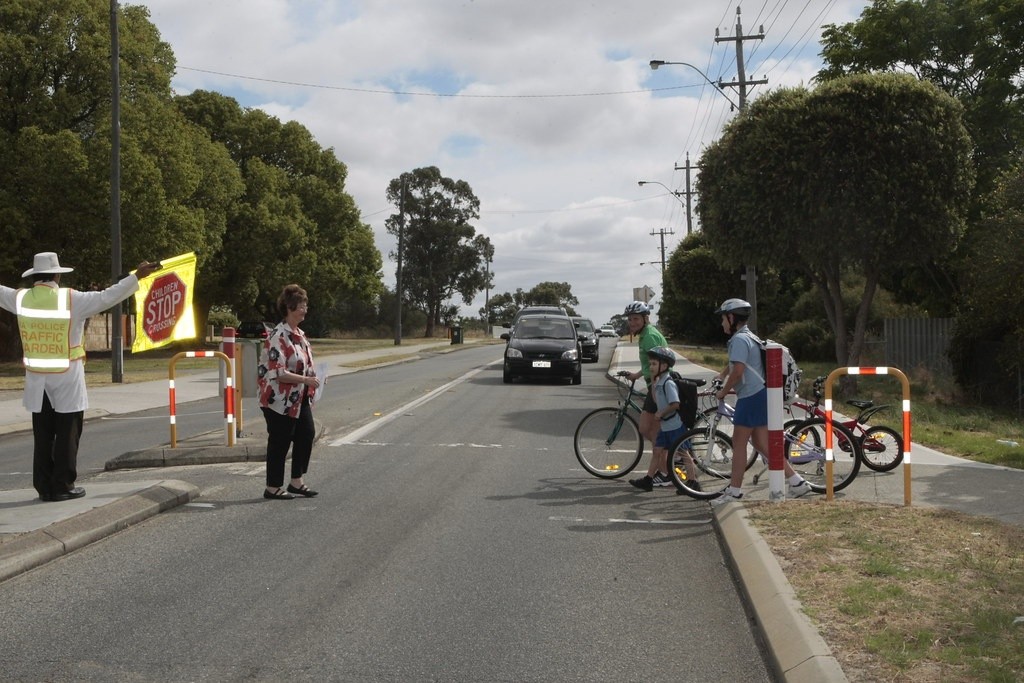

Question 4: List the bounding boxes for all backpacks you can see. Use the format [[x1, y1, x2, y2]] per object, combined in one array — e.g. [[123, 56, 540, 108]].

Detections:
[[663, 377, 697, 429], [742, 331, 802, 405]]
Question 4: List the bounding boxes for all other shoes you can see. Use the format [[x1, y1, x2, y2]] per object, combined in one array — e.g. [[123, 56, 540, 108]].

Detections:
[[264, 487, 292, 499], [287, 484, 318, 496]]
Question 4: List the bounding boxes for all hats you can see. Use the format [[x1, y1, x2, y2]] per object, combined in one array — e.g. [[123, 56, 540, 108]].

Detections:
[[22, 252, 73, 277]]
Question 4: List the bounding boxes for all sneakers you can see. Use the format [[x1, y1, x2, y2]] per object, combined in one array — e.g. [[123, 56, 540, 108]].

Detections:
[[653, 471, 673, 486], [675, 458, 697, 467], [675, 479, 700, 494], [787, 480, 811, 498], [628, 476, 653, 491], [709, 487, 743, 507]]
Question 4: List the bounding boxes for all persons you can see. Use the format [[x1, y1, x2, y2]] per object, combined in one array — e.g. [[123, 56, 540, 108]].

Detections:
[[624, 301, 674, 486], [709, 298, 811, 504], [0, 252, 158, 502], [629, 346, 701, 495], [257, 285, 319, 499]]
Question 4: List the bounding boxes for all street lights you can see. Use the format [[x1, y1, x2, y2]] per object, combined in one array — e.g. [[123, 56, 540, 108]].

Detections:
[[640, 262, 667, 301], [649, 59, 758, 339], [637, 181, 693, 235]]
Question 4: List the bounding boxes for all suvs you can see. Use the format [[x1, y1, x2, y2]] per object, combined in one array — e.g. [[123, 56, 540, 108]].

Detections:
[[501, 303, 580, 336]]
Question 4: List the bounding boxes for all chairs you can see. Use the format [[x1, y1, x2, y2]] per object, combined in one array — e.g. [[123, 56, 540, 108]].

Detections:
[[580, 323, 588, 331], [523, 325, 570, 336]]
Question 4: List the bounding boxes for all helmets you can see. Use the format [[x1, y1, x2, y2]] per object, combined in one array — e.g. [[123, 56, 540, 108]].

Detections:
[[715, 298, 752, 316], [623, 300, 650, 316], [645, 346, 675, 368]]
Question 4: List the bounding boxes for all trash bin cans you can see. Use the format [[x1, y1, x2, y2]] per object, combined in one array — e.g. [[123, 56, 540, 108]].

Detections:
[[448, 326, 464, 345]]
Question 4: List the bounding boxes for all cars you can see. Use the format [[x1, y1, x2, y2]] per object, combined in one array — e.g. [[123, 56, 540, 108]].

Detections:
[[600, 322, 616, 337], [569, 316, 602, 363], [500, 313, 587, 385]]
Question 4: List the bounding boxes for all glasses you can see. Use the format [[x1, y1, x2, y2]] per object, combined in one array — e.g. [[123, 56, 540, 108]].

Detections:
[[289, 306, 309, 313]]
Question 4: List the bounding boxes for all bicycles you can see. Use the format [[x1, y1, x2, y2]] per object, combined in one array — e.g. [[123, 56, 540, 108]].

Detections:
[[784, 373, 904, 472], [573, 370, 760, 480], [664, 378, 862, 502]]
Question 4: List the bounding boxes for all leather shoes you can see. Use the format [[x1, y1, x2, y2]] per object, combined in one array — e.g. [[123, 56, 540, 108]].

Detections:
[[38, 493, 54, 501], [55, 487, 86, 500]]
